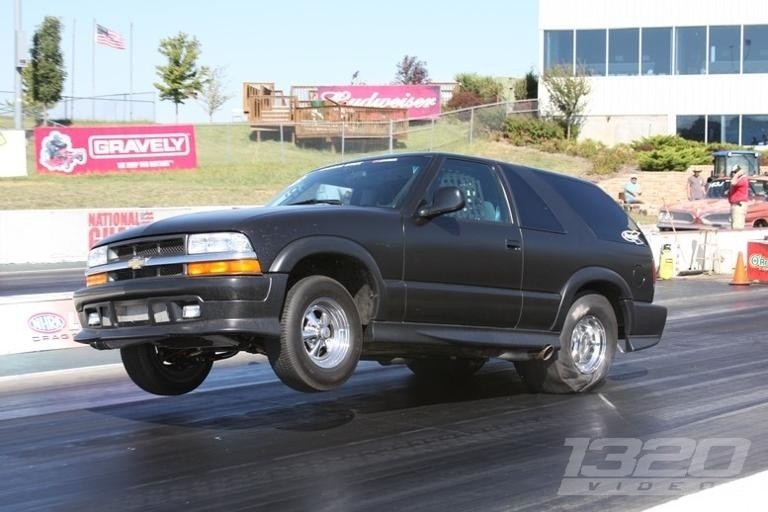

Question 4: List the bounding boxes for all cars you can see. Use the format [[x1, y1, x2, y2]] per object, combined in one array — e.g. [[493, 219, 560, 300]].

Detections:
[[653, 178, 767, 236]]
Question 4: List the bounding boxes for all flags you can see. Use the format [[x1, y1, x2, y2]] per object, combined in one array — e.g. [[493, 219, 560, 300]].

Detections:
[[94, 21, 129, 52]]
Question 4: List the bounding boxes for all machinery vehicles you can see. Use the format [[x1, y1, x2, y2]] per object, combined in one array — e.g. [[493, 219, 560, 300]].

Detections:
[[701, 147, 765, 199]]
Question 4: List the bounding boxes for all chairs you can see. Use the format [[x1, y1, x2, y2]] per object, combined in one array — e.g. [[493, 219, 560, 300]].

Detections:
[[438, 165, 483, 219]]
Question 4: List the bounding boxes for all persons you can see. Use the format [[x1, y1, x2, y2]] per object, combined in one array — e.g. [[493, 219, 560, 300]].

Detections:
[[685, 166, 707, 202], [726, 163, 750, 232], [309, 92, 324, 122], [623, 173, 655, 212]]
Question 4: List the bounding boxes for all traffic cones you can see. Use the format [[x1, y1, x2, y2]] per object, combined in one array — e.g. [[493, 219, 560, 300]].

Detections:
[[728, 249, 754, 288]]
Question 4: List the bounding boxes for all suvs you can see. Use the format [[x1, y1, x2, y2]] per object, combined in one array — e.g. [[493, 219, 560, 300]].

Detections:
[[69, 150, 668, 401]]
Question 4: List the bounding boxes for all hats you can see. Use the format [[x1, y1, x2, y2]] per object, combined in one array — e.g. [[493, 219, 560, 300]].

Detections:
[[692, 167, 702, 172], [631, 174, 637, 178]]
